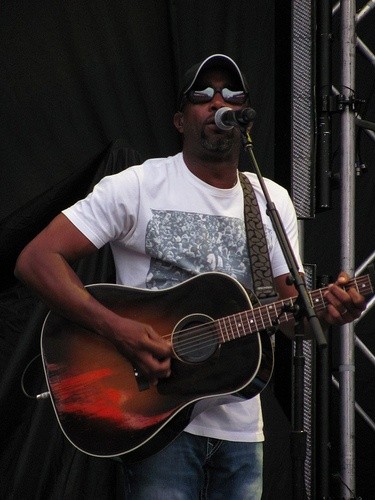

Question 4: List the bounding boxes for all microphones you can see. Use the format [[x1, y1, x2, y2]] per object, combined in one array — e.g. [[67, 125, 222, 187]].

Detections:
[[214, 106, 256, 131]]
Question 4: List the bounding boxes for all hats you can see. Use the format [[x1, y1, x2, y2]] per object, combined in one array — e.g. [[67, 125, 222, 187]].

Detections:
[[179, 49, 250, 101]]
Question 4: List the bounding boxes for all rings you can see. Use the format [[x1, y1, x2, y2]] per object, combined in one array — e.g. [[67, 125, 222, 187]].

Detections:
[[341, 309, 348, 315]]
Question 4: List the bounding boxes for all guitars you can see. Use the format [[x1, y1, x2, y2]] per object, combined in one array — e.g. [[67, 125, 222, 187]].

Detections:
[[40, 271, 375, 462]]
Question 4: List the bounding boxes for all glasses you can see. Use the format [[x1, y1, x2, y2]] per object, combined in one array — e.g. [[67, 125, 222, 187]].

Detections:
[[179, 83, 250, 111]]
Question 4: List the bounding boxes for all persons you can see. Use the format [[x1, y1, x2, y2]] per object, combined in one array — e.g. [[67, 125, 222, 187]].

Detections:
[[14, 52, 367, 500]]
